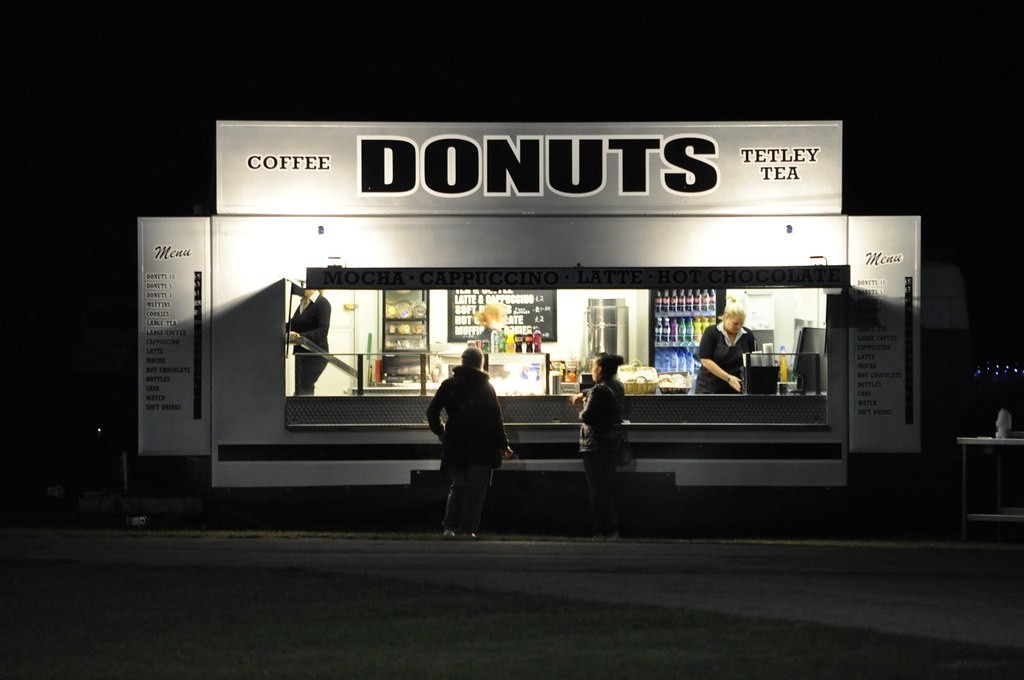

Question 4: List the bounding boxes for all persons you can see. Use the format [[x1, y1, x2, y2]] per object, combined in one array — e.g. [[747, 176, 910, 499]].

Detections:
[[571, 352, 626, 542], [286, 289, 331, 395], [692, 295, 759, 394], [425, 348, 514, 538]]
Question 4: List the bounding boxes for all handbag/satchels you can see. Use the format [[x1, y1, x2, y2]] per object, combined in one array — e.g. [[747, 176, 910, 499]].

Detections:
[[600, 381, 633, 466]]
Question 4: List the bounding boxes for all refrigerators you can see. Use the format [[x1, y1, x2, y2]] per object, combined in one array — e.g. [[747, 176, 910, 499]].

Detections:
[[649, 287, 726, 395]]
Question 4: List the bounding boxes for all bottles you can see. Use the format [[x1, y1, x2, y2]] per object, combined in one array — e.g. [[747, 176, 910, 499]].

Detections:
[[655, 316, 716, 342], [526, 326, 533, 352], [533, 328, 542, 353], [514, 328, 523, 352], [490, 329, 498, 353], [655, 348, 703, 374], [506, 328, 515, 353], [498, 328, 506, 353], [655, 289, 714, 313]]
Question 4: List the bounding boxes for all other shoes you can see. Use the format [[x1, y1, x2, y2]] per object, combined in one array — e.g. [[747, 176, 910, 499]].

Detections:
[[587, 533, 624, 544], [441, 529, 480, 542]]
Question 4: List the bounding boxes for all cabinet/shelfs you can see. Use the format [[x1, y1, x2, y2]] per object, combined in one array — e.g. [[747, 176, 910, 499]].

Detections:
[[382, 290, 430, 378]]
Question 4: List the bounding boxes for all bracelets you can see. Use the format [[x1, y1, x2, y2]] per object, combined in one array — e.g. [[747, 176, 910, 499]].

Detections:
[[727, 375, 731, 383]]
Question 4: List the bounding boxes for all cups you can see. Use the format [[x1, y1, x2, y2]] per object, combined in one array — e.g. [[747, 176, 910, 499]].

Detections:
[[742, 344, 775, 367]]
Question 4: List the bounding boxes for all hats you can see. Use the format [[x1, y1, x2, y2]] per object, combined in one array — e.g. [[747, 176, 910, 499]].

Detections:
[[599, 354, 624, 376]]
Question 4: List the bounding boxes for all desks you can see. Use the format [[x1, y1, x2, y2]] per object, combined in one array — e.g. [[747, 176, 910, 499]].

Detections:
[[956, 436, 1024, 542]]
